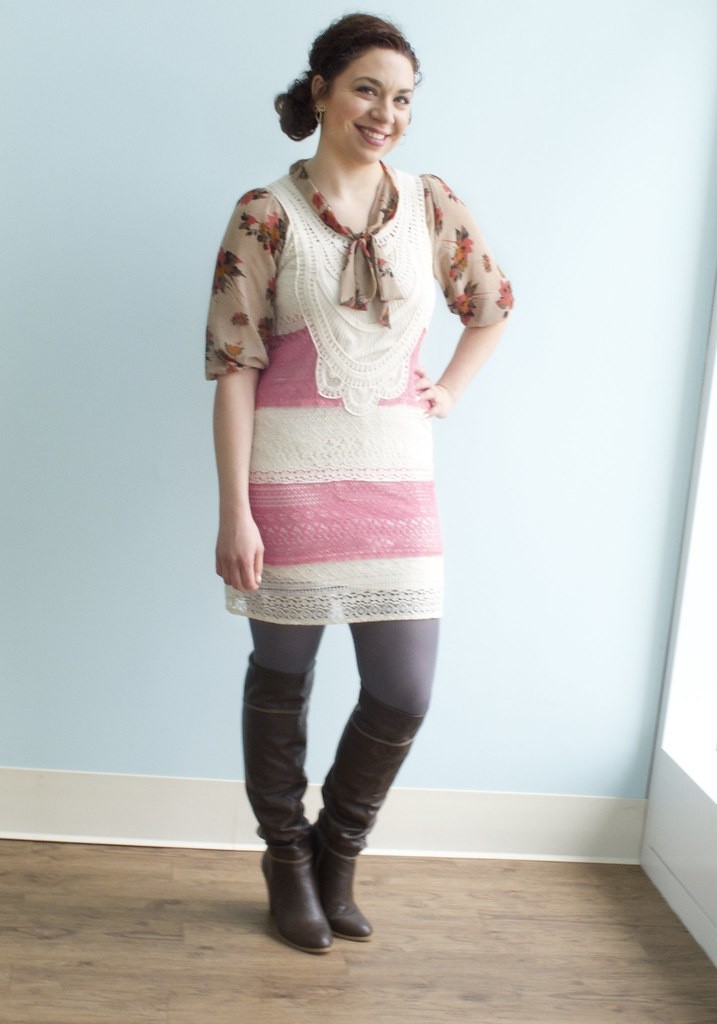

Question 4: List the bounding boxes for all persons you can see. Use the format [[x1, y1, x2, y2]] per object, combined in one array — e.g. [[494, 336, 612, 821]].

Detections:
[[204, 15, 516, 954]]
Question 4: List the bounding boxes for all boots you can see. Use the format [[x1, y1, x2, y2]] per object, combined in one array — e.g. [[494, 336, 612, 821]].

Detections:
[[309, 684, 424, 940], [242, 651, 333, 952]]
